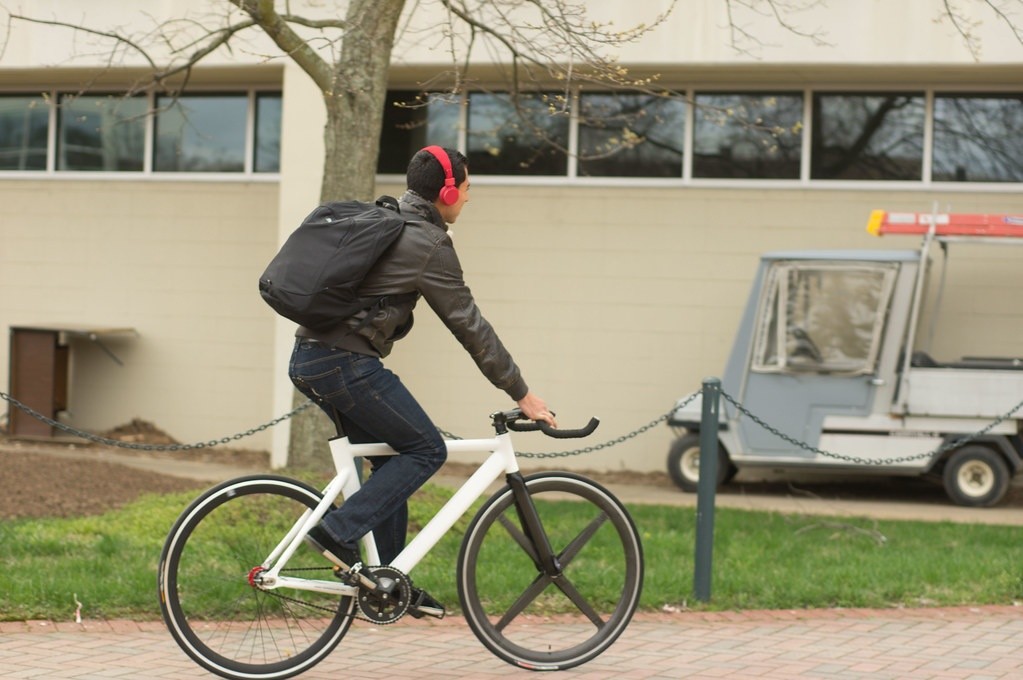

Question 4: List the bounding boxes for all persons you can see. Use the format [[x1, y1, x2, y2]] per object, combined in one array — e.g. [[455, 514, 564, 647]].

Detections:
[[289, 146, 557, 615]]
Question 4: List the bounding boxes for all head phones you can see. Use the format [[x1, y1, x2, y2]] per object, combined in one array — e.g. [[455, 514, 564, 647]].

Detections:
[[422, 145, 460, 205]]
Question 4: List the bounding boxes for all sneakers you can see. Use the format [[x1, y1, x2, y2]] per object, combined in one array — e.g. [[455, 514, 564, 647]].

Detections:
[[393, 575, 445, 615], [307, 525, 377, 589]]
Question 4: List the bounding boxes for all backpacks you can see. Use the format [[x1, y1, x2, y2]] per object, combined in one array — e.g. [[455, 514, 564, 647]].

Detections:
[[259, 194, 428, 332]]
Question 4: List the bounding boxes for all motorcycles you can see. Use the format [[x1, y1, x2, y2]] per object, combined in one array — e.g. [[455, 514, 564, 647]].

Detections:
[[667, 211, 1023, 509]]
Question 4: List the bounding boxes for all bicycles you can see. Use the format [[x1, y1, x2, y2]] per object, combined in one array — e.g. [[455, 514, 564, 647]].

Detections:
[[157, 374, 645, 680]]
